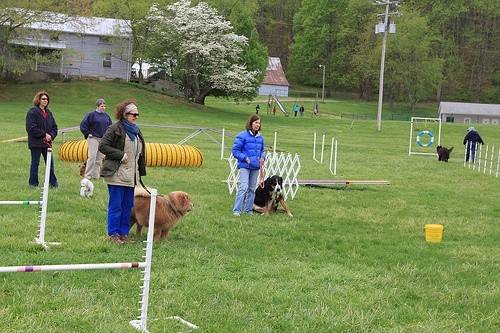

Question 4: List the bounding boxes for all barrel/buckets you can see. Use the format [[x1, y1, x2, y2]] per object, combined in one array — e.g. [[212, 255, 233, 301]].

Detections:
[[425, 224, 442, 243]]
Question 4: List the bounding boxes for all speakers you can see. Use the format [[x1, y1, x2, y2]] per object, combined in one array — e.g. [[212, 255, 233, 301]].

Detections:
[[375, 23, 386, 34], [389, 24, 396, 34]]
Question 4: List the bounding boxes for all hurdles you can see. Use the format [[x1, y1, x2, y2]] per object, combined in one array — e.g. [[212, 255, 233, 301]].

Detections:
[[0, 148, 62, 252], [0, 188, 199, 333]]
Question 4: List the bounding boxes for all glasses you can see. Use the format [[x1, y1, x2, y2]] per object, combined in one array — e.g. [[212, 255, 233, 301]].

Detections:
[[42, 98, 48, 100], [131, 114, 139, 116]]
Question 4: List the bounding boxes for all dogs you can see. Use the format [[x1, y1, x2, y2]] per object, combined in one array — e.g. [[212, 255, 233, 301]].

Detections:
[[129, 191, 194, 244], [243, 175, 293, 217], [436, 145, 453, 162], [80, 178, 94, 198]]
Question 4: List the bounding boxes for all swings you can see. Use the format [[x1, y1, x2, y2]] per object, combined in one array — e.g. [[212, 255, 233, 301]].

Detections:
[[283, 92, 311, 117]]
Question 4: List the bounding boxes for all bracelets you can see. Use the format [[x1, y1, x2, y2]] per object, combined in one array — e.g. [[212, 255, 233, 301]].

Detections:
[[246, 158, 249, 161]]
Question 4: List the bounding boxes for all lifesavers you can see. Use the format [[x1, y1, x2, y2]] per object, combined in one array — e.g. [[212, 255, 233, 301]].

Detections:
[[416, 130, 434, 147]]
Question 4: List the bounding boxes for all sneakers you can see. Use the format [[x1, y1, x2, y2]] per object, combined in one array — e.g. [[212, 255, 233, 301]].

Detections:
[[109, 235, 125, 243], [118, 235, 136, 243]]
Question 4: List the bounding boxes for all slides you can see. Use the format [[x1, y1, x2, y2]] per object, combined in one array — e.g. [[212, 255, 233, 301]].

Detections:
[[271, 94, 287, 114]]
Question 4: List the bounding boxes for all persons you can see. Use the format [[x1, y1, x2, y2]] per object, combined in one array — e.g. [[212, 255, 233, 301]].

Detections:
[[294, 105, 298, 117], [26, 91, 58, 189], [232, 114, 265, 217], [80, 98, 113, 179], [299, 104, 304, 117], [256, 104, 260, 114], [273, 104, 276, 115], [98, 99, 146, 245], [463, 127, 484, 163]]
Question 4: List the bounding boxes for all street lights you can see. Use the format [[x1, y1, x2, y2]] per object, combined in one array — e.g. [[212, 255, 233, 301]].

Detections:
[[318, 64, 326, 103]]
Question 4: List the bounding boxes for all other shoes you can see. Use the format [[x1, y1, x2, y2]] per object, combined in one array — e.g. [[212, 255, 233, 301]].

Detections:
[[234, 213, 240, 216]]
